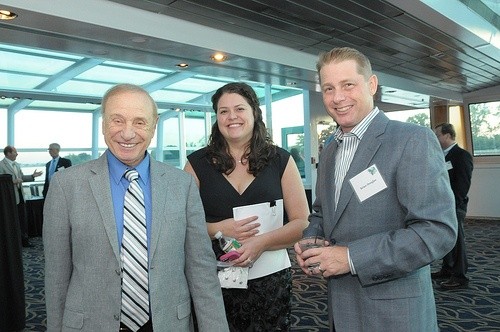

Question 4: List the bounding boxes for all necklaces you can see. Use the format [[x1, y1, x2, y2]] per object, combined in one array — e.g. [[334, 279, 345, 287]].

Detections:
[[236, 157, 247, 163]]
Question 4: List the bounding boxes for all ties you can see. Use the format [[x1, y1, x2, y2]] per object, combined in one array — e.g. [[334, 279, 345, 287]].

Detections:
[[13, 163, 20, 188], [49, 160, 56, 181], [120, 167, 150, 332]]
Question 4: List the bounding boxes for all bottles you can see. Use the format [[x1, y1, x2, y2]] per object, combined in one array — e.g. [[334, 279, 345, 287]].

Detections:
[[214, 230, 241, 254]]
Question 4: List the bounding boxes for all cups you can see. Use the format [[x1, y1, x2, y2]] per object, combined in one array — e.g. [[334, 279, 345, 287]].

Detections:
[[298, 236, 325, 271]]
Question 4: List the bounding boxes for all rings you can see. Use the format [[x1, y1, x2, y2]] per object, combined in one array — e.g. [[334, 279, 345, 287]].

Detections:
[[247, 258, 253, 264], [318, 265, 323, 273]]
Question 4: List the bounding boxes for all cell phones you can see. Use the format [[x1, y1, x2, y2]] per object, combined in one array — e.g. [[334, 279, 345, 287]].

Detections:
[[220, 251, 242, 262]]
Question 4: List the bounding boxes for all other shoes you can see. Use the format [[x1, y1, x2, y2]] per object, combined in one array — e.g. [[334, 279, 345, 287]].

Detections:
[[440, 276, 468, 289], [430, 270, 453, 279]]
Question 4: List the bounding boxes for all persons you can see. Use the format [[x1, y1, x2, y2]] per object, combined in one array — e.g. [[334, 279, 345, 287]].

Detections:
[[430, 122, 474, 287], [0, 146, 43, 247], [183, 82, 311, 332], [294, 47, 458, 332], [42, 142, 72, 199], [42, 83, 230, 332]]
